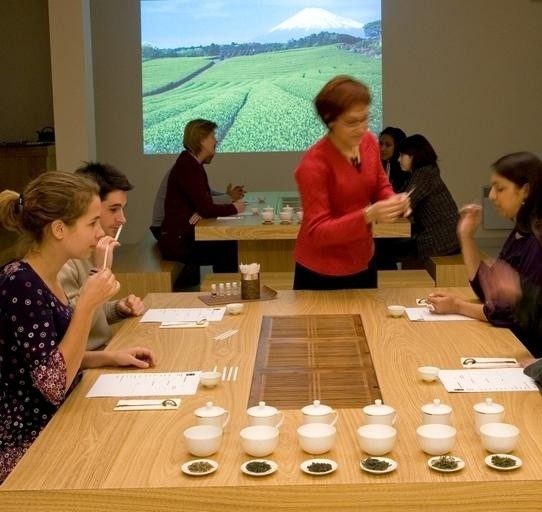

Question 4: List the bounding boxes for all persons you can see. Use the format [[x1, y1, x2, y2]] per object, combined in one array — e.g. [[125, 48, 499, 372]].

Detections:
[[56, 163, 146, 351], [2, 171, 156, 483], [482, 194, 541, 389], [293, 76, 412, 290], [425, 151, 542, 327], [150, 141, 247, 265], [156, 120, 245, 273], [377, 127, 406, 196], [390, 134, 464, 263]]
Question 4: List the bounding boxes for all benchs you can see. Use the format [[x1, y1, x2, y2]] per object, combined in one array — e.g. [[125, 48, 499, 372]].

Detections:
[[107, 239, 185, 303], [197, 265, 434, 295], [424, 247, 496, 289]]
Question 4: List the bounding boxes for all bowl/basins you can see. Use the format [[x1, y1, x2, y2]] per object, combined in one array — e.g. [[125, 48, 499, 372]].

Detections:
[[417, 422, 458, 454], [239, 425, 279, 457], [296, 423, 337, 455], [184, 425, 223, 457], [480, 422, 520, 453], [356, 424, 398, 457]]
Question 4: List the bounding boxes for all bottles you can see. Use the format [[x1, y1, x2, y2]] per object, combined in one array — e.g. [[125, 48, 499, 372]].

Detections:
[[210, 281, 242, 298]]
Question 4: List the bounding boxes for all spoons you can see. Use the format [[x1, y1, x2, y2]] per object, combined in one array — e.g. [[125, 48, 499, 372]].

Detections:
[[462, 358, 515, 365], [117, 399, 177, 406], [163, 319, 207, 325]]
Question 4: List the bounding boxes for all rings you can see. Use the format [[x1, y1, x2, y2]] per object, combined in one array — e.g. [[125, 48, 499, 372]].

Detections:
[[428, 303, 434, 312]]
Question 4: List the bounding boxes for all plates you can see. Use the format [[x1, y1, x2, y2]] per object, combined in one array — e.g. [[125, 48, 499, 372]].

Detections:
[[240, 460, 278, 477], [359, 457, 397, 475], [301, 458, 337, 476], [485, 453, 522, 472], [181, 458, 218, 476], [427, 455, 465, 472]]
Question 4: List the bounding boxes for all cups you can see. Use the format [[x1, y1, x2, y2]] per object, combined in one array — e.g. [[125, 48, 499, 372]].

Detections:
[[472, 396, 505, 437], [362, 399, 398, 426], [251, 205, 302, 224], [197, 371, 219, 389], [225, 303, 244, 315], [195, 402, 230, 429], [247, 402, 284, 429], [417, 366, 440, 382], [300, 399, 339, 427], [421, 398, 452, 424], [387, 305, 405, 317]]
